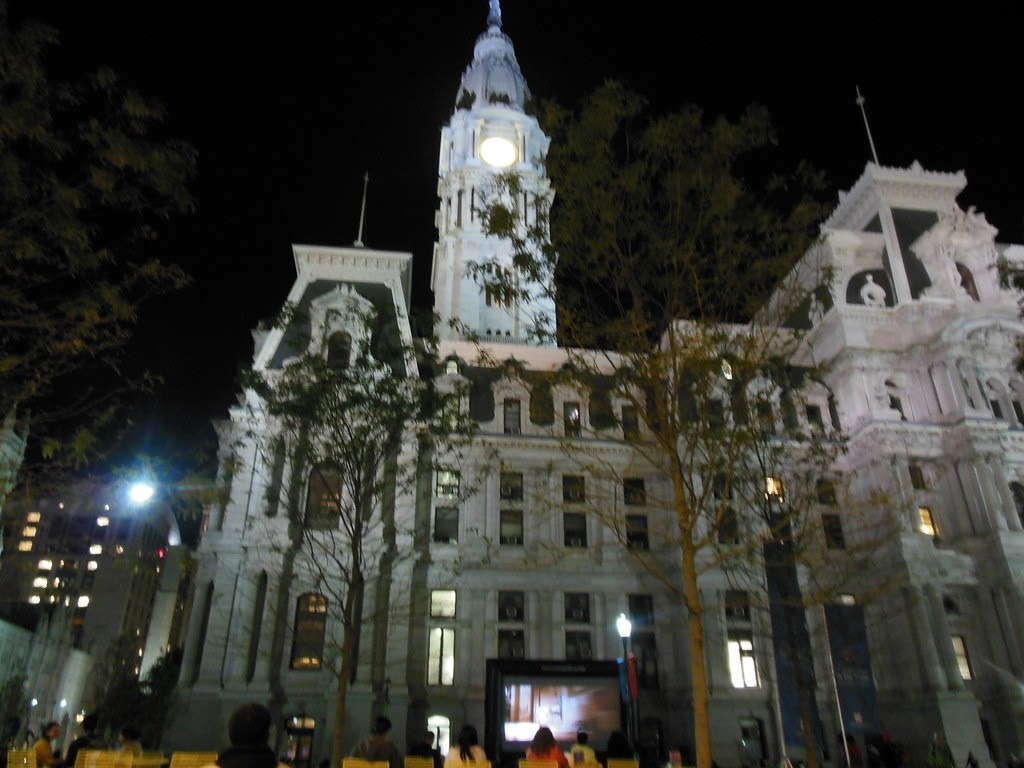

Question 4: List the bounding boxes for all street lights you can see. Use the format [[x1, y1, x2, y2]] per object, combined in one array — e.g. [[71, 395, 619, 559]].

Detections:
[[617, 611, 636, 754]]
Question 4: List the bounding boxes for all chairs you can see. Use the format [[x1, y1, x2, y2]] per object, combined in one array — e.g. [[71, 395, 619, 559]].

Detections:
[[444, 761, 492, 768], [519, 759, 558, 768], [608, 759, 639, 768], [404, 756, 434, 768], [7, 749, 219, 768], [343, 757, 389, 768]]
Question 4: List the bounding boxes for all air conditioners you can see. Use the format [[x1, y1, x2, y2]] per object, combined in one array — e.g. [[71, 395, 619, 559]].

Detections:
[[443, 485, 453, 493], [501, 427, 745, 657], [749, 727, 760, 738]]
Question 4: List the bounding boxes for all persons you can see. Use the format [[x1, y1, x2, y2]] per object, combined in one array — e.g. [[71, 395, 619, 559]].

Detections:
[[352, 716, 404, 768], [120, 724, 143, 759], [526, 727, 570, 768], [860, 274, 886, 308], [30, 722, 64, 768], [928, 731, 957, 768], [201, 702, 290, 768], [410, 732, 443, 768], [606, 731, 633, 758], [444, 726, 491, 768], [966, 752, 979, 768], [809, 292, 824, 327], [63, 714, 105, 768], [838, 734, 864, 768], [571, 732, 597, 765]]
